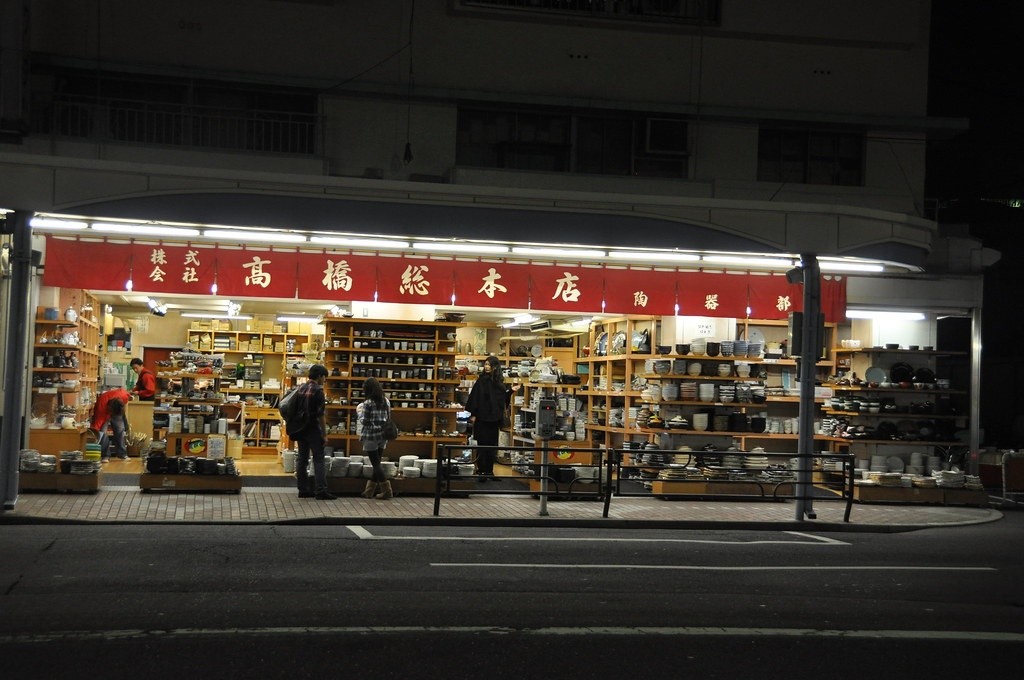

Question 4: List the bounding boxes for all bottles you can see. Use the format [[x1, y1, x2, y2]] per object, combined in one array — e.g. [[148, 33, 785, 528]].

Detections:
[[36, 351, 79, 368], [327, 367, 459, 380]]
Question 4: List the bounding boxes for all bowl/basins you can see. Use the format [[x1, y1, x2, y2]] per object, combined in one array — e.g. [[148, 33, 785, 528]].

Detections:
[[923, 346, 933, 350], [641, 382, 767, 404], [566, 419, 585, 441], [658, 340, 762, 358], [841, 340, 860, 349], [635, 408, 820, 434], [886, 344, 899, 349], [645, 360, 760, 378], [909, 346, 919, 350], [821, 380, 954, 440]]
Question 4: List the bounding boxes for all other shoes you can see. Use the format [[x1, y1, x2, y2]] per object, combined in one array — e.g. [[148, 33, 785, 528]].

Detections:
[[123, 455, 132, 461], [299, 490, 315, 498], [101, 456, 110, 463], [315, 493, 338, 500], [478, 472, 502, 483]]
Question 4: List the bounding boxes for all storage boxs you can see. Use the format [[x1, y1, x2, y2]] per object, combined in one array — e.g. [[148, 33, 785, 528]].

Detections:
[[189, 319, 310, 352], [86, 443, 101, 460]]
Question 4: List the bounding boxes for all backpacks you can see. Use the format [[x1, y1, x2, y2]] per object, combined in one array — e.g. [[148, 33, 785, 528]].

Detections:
[[286, 385, 324, 441]]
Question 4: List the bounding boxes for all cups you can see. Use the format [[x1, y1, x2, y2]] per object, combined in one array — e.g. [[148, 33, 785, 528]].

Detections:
[[329, 382, 454, 408], [334, 353, 431, 364], [354, 330, 383, 338], [65, 309, 76, 321], [354, 340, 455, 352], [333, 341, 340, 347]]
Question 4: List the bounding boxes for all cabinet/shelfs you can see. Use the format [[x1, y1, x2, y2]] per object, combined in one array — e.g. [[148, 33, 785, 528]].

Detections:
[[187, 329, 310, 455], [317, 314, 968, 477], [29, 287, 101, 471], [153, 366, 185, 441], [166, 374, 227, 458]]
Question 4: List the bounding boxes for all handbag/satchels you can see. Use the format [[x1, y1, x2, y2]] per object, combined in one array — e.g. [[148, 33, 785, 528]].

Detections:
[[384, 418, 398, 441], [500, 403, 511, 430], [277, 386, 299, 422]]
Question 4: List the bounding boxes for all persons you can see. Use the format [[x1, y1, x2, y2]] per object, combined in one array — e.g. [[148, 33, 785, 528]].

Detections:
[[130, 358, 155, 401], [465, 358, 526, 481], [91, 388, 132, 462], [356, 378, 394, 498], [296, 365, 337, 500]]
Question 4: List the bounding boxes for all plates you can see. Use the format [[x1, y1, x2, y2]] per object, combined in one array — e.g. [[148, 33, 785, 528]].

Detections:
[[635, 440, 768, 469], [20, 449, 102, 474], [887, 457, 904, 472], [309, 447, 475, 479], [147, 451, 239, 475], [889, 362, 914, 381], [914, 369, 933, 381], [865, 367, 886, 382], [854, 466, 941, 474], [531, 344, 542, 357]]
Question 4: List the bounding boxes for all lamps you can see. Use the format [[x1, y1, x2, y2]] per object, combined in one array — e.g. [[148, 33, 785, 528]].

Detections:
[[562, 316, 593, 328], [495, 318, 519, 328]]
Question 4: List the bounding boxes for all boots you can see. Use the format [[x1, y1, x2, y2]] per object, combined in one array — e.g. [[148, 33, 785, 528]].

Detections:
[[376, 480, 393, 499], [360, 480, 378, 499]]
